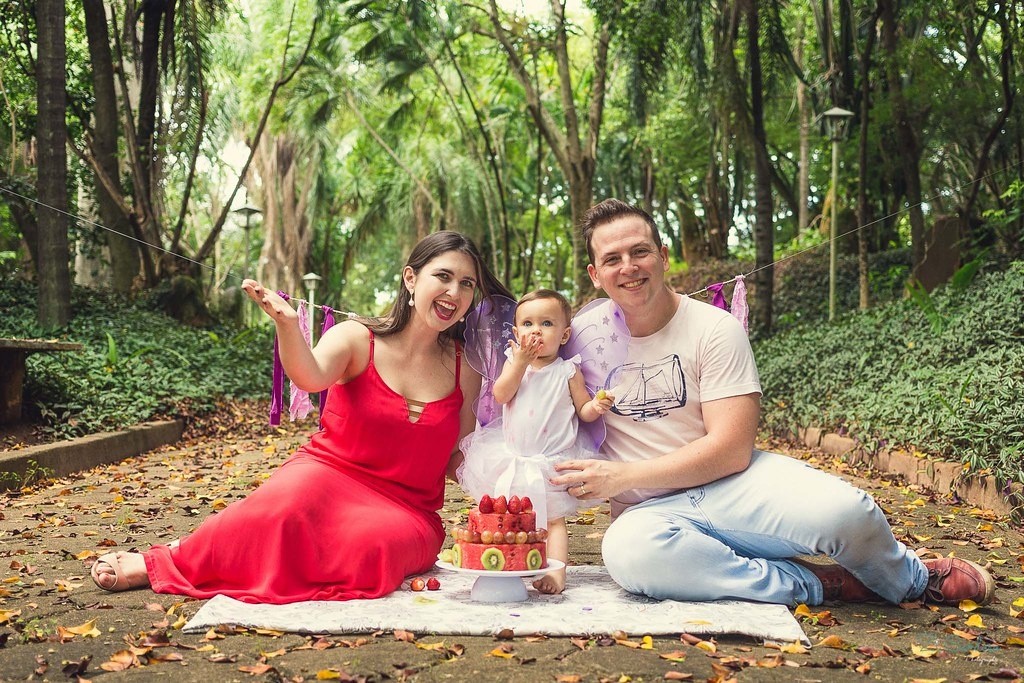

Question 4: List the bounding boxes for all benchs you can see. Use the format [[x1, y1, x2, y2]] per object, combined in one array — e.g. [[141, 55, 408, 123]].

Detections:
[[0, 339, 84, 424]]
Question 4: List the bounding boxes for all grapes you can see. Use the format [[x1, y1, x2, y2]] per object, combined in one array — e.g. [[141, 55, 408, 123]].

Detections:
[[596, 389, 607, 399]]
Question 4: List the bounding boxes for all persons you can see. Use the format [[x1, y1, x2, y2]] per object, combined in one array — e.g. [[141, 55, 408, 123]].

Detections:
[[549, 197, 995, 608], [92, 229, 518, 604], [455, 288, 616, 594]]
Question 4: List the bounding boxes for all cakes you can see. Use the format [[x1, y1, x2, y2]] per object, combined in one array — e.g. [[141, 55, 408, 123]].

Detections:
[[450, 494, 548, 571]]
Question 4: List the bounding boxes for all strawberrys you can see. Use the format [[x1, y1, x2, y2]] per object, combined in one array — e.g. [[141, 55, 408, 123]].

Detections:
[[411, 577, 425, 591], [426, 577, 440, 590]]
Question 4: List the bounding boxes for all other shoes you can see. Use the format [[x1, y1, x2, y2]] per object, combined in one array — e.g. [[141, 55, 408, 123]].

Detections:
[[793, 557, 874, 602], [918, 557, 995, 606]]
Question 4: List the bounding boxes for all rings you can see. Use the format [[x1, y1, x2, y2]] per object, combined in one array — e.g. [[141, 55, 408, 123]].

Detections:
[[581, 484, 586, 494]]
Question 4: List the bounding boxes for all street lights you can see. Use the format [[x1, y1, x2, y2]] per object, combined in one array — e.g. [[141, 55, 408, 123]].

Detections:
[[302, 271, 322, 350], [235, 205, 264, 328], [822, 103, 853, 320]]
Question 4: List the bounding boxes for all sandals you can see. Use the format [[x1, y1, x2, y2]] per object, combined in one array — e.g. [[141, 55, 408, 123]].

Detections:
[[163, 540, 182, 548], [90, 551, 152, 591]]
